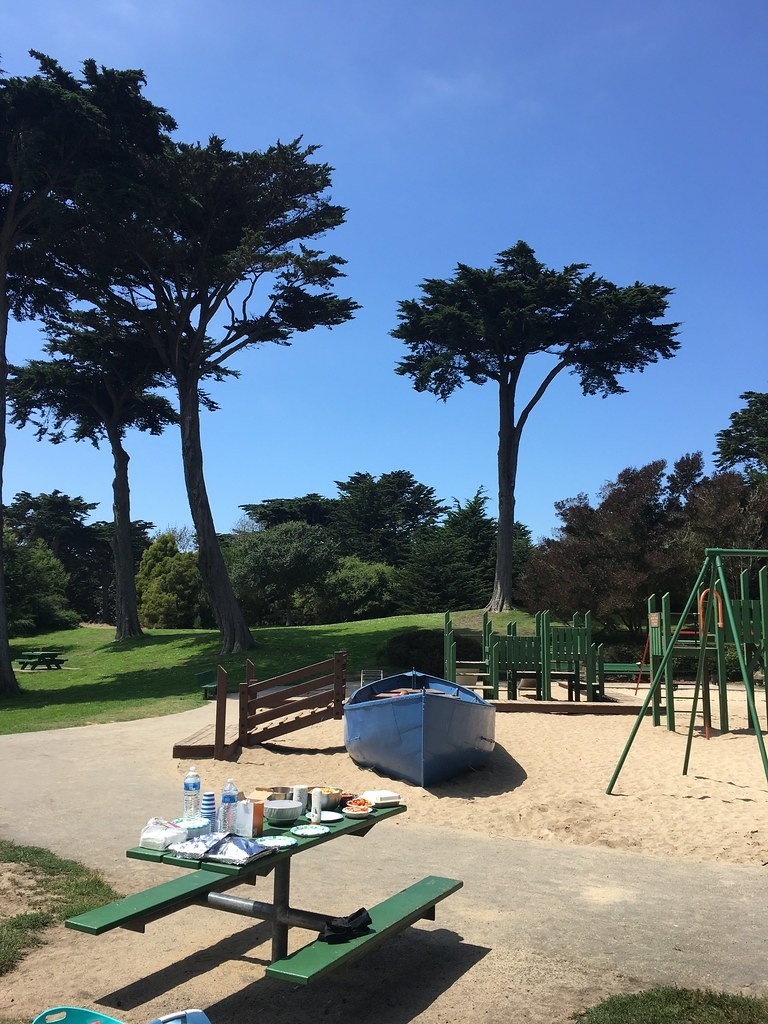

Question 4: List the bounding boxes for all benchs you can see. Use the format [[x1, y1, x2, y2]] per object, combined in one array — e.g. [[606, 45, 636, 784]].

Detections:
[[264, 874, 462, 986], [201, 682, 218, 699], [19, 658, 68, 670], [595, 663, 650, 683], [65, 872, 259, 936]]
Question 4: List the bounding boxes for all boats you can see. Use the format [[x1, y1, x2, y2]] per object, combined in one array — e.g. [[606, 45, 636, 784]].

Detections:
[[343, 667, 497, 787]]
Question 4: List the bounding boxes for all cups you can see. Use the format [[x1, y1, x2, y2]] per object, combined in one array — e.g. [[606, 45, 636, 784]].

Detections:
[[200, 792, 216, 832], [217, 807, 223, 832]]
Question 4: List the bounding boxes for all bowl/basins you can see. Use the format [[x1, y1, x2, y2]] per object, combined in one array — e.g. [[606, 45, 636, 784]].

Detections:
[[307, 786, 343, 811], [342, 807, 373, 819], [340, 794, 357, 811], [346, 800, 375, 808], [293, 786, 308, 815], [267, 787, 295, 800], [264, 799, 302, 818]]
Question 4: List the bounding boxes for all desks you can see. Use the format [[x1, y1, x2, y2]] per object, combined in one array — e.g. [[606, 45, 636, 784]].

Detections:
[[126, 803, 406, 962], [21, 652, 62, 670]]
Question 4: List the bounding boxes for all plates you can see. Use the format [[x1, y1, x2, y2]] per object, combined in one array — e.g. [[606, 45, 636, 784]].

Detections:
[[374, 801, 400, 808], [172, 817, 212, 839], [290, 825, 330, 837], [266, 818, 297, 826], [255, 835, 297, 849], [306, 811, 344, 822]]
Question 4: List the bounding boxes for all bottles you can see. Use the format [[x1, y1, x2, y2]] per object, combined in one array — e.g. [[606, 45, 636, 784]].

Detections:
[[222, 778, 238, 833], [183, 766, 200, 824]]
